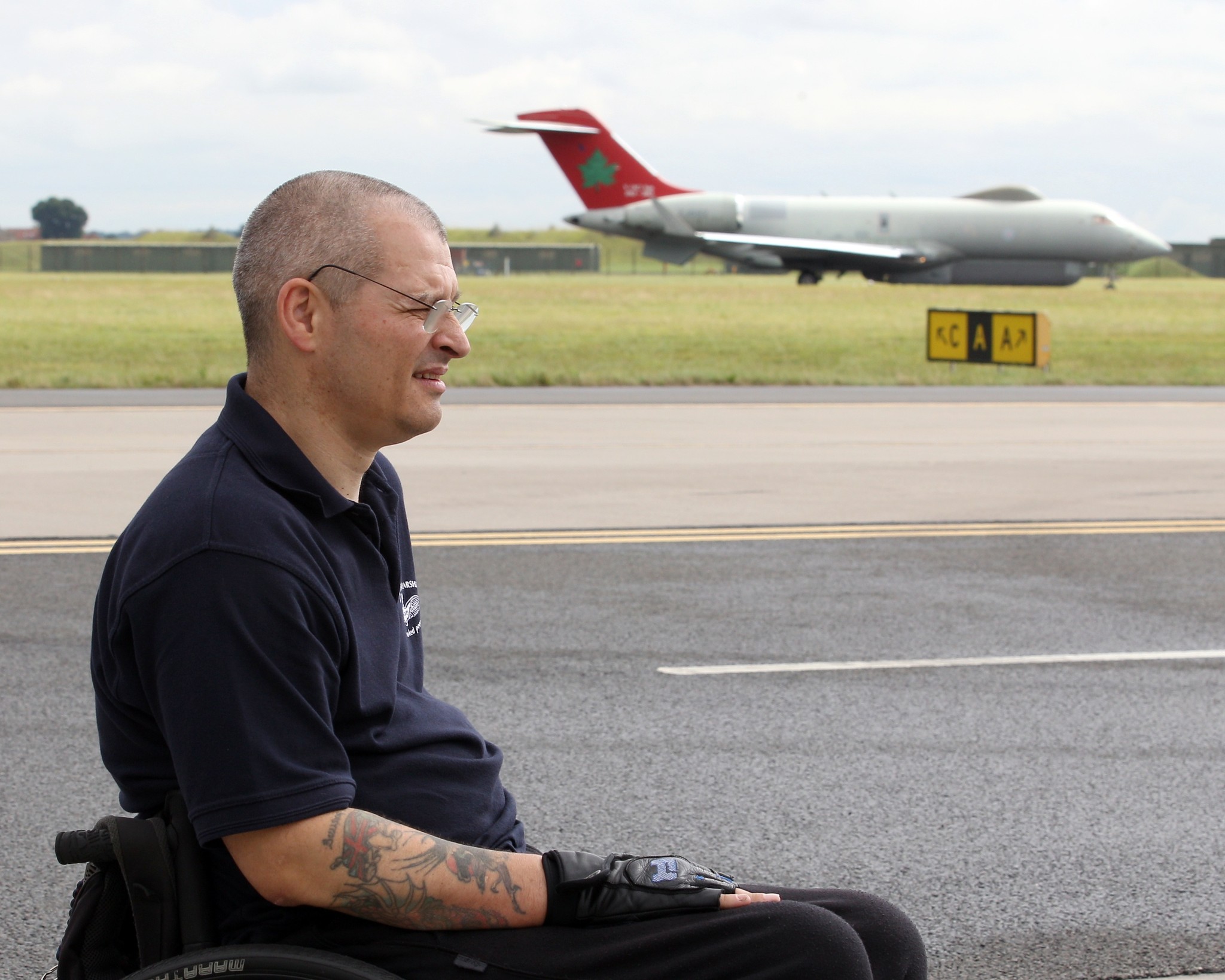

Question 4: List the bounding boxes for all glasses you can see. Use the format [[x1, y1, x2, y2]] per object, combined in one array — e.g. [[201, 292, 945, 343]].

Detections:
[[306, 263, 479, 333]]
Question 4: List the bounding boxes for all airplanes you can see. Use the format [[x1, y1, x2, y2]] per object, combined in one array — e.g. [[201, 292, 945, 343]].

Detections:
[[487, 110, 1173, 288]]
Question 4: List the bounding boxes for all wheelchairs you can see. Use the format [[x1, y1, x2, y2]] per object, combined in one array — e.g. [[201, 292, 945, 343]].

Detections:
[[54, 811, 430, 980]]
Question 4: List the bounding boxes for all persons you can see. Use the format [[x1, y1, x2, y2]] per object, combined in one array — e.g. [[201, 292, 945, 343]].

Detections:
[[89, 171, 930, 980]]
